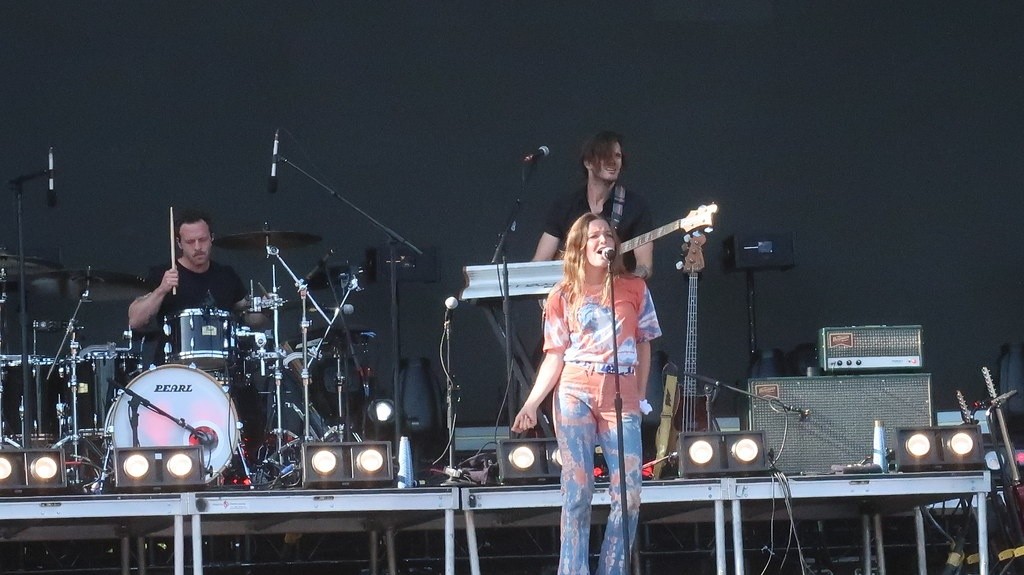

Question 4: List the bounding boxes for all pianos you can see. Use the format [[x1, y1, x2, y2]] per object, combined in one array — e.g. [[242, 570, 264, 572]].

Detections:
[[454, 257, 581, 437]]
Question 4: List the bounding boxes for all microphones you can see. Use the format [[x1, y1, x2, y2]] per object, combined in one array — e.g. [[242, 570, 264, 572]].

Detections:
[[48, 147, 56, 208], [443, 297, 459, 329], [798, 410, 810, 422], [523, 145, 549, 161], [602, 248, 616, 261], [305, 249, 335, 279], [310, 303, 353, 315], [269, 130, 280, 185], [200, 433, 214, 445]]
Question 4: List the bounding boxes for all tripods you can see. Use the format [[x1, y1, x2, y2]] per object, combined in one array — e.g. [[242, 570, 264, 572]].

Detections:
[[247, 245, 364, 483], [46, 278, 114, 488]]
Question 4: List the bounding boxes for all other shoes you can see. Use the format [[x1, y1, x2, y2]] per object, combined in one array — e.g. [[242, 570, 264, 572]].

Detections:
[[594, 468, 602, 477], [642, 466, 652, 476]]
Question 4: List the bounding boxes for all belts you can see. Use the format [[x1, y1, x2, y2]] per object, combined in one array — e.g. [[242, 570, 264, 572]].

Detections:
[[566, 360, 635, 375]]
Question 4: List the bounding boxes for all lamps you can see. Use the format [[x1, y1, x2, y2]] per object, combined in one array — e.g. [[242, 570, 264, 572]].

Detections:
[[112, 443, 209, 491], [495, 434, 561, 483], [676, 429, 770, 477], [889, 422, 985, 470], [300, 438, 395, 488], [0, 446, 68, 492], [368, 398, 394, 441]]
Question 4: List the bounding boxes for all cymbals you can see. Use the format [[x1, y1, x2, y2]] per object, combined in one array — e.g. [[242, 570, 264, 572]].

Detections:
[[230, 297, 312, 314], [293, 257, 373, 290], [26, 262, 149, 303], [0, 253, 65, 282], [211, 230, 322, 250]]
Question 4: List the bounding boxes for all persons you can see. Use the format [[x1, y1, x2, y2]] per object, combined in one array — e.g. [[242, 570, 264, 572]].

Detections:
[[127, 209, 267, 487], [527, 129, 655, 487], [511, 213, 664, 574]]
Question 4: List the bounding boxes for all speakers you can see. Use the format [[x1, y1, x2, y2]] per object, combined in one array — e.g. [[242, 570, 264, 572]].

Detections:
[[748, 372, 934, 475]]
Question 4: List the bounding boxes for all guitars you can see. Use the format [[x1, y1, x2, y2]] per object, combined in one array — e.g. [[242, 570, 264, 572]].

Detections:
[[654, 231, 723, 481], [612, 203, 720, 255], [953, 366, 1024, 540]]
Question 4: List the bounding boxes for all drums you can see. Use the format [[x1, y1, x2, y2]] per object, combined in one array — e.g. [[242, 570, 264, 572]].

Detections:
[[161, 307, 236, 374], [224, 327, 275, 374], [64, 353, 143, 439], [104, 363, 240, 484], [1, 357, 68, 442]]
